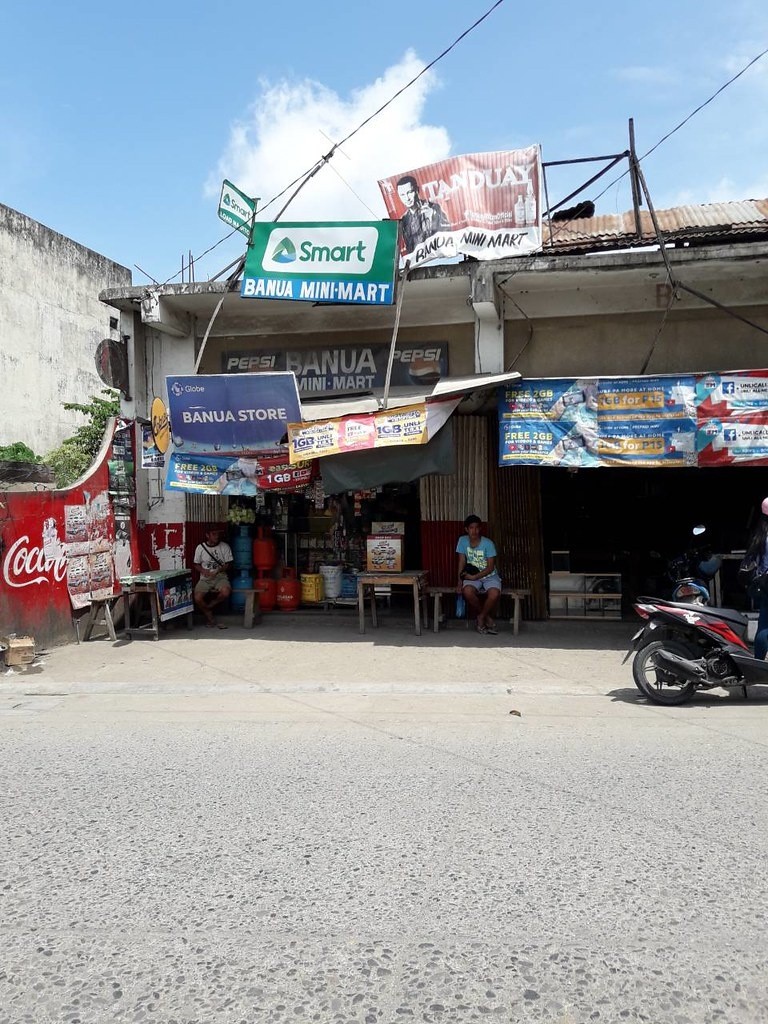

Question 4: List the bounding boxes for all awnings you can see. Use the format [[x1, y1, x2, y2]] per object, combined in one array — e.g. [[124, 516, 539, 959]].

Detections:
[[155, 372, 521, 497]]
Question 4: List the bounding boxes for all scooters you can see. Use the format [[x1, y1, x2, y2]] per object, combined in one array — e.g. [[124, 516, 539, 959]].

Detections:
[[621, 568, 768, 706], [649, 524, 710, 606]]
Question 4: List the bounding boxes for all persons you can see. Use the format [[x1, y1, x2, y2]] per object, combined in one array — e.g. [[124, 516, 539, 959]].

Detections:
[[456, 515, 502, 634], [739, 496, 768, 660], [194, 526, 233, 629]]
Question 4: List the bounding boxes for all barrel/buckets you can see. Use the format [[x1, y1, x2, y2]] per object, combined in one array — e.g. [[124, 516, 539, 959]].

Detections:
[[301, 573, 323, 603], [319, 566, 343, 597]]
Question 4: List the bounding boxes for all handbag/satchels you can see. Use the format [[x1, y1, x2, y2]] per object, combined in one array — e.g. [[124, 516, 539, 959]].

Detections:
[[456, 594, 466, 619]]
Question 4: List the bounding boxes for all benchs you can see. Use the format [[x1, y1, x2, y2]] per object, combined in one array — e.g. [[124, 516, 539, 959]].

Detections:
[[207, 588, 267, 627], [424, 587, 533, 637], [84, 592, 134, 642]]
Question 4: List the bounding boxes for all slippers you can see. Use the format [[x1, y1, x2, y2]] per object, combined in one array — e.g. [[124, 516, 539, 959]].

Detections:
[[206, 618, 228, 630], [476, 622, 498, 635]]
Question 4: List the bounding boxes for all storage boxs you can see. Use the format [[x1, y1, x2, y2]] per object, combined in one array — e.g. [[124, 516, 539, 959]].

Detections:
[[341, 574, 357, 598], [366, 521, 406, 573]]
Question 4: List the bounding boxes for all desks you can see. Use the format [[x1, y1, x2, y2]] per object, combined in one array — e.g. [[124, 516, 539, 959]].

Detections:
[[120, 568, 193, 641], [356, 570, 430, 636]]
